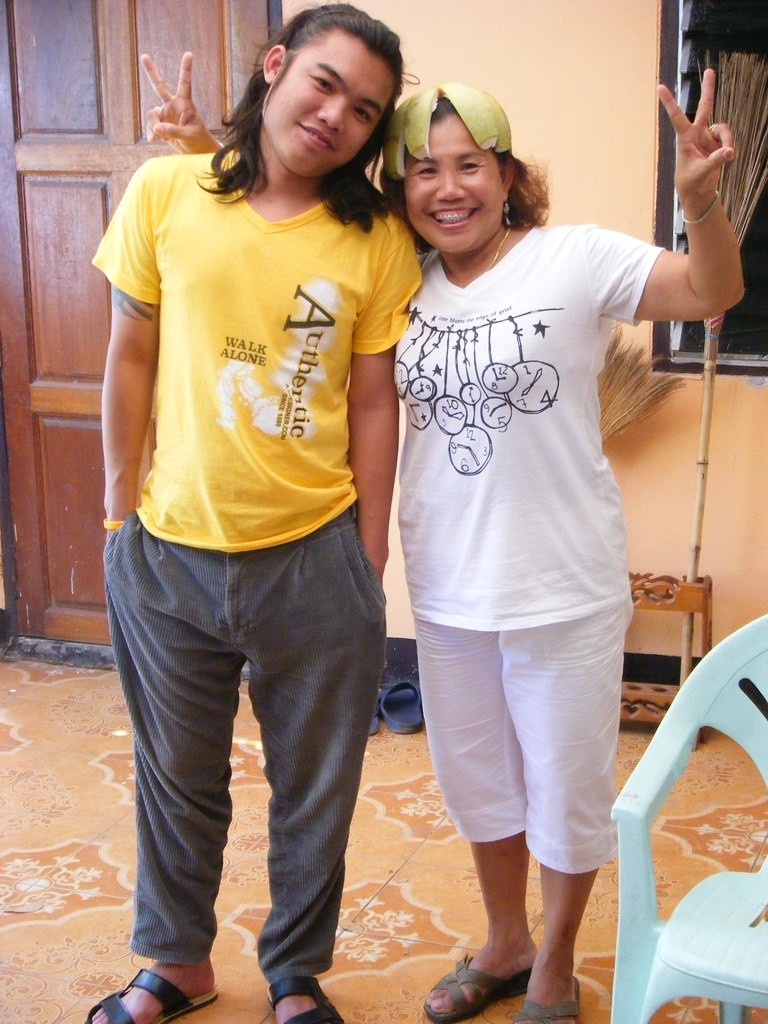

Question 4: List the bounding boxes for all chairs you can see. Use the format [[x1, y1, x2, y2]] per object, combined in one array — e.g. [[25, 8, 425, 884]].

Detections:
[[610, 613, 768, 1024]]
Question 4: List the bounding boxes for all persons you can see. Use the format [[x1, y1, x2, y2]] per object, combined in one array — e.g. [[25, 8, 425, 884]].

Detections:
[[86, 5, 422, 1024], [139, 51, 744, 1024]]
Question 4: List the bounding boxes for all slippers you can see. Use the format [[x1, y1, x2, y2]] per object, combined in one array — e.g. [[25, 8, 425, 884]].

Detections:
[[265, 976, 346, 1024], [421, 954, 531, 1023], [85, 968, 220, 1024], [510, 975, 581, 1023], [368, 691, 381, 736], [377, 681, 423, 734]]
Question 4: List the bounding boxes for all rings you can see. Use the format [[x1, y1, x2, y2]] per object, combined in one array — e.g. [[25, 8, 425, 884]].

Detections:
[[708, 123, 721, 134]]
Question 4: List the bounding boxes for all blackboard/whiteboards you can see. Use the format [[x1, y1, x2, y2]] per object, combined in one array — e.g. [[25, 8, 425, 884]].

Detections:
[[650, 0, 768, 379]]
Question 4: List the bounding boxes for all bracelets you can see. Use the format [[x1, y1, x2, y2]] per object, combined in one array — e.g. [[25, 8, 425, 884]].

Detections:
[[679, 190, 721, 224], [102, 519, 124, 531]]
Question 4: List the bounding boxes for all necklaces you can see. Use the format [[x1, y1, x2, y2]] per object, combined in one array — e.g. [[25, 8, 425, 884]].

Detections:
[[488, 227, 510, 272]]
